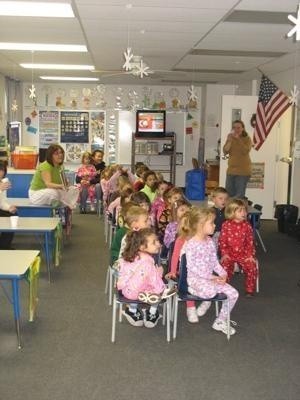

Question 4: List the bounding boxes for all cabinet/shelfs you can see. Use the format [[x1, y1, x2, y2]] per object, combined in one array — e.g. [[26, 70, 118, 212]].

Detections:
[[131, 132, 176, 187]]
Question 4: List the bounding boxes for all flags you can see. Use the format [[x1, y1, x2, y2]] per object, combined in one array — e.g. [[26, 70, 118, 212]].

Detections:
[[252, 75, 293, 151]]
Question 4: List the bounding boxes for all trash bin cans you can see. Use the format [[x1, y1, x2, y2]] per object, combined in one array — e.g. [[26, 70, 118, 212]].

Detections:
[[274, 205, 298, 233]]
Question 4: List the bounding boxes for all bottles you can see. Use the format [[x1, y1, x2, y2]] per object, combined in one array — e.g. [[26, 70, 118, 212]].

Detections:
[[61, 183, 82, 208]]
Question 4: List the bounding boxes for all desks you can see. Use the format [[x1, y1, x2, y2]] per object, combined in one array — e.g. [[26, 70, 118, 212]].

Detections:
[[0, 167, 73, 353]]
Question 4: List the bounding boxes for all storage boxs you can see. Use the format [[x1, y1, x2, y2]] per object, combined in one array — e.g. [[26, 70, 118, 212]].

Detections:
[[11, 153, 40, 169]]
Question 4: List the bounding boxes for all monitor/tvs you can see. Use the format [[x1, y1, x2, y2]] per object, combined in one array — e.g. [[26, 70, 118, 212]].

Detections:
[[135, 109, 167, 137]]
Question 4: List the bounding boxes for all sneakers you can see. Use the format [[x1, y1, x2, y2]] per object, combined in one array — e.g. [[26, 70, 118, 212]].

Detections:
[[246, 292, 254, 297], [186, 307, 199, 323], [142, 308, 150, 326], [211, 318, 236, 336], [81, 205, 96, 215], [197, 301, 212, 317], [122, 307, 144, 327], [145, 311, 160, 328]]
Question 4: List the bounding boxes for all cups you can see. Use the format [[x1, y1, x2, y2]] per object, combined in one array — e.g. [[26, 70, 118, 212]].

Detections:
[[2, 177, 11, 189], [11, 216, 19, 227]]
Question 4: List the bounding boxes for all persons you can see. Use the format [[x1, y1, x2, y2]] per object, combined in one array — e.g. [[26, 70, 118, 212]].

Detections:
[[0, 159, 19, 250], [75, 148, 260, 336], [223, 120, 252, 200], [28, 144, 70, 206]]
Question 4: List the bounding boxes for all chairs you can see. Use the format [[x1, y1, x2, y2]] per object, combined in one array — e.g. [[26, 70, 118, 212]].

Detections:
[[75, 157, 269, 344]]
